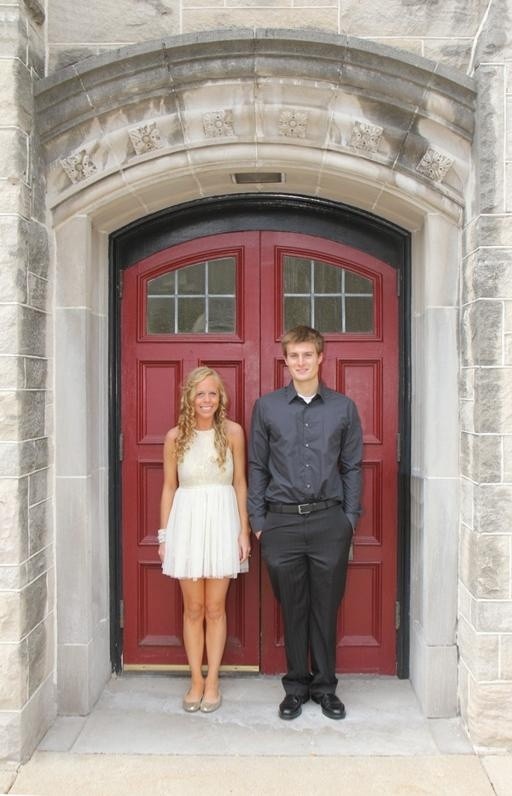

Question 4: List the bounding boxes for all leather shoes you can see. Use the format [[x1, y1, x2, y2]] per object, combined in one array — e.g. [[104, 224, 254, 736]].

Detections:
[[199, 690, 221, 712], [183, 687, 203, 712], [279, 694, 310, 719], [311, 691, 346, 718]]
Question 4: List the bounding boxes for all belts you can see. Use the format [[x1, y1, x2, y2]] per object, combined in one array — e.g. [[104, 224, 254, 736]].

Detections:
[[268, 499, 341, 515]]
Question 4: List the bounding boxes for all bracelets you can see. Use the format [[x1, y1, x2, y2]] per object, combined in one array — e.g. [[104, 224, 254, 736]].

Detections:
[[156, 527, 168, 545]]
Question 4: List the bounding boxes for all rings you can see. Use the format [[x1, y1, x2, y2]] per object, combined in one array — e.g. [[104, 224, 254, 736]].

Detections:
[[248, 554, 252, 558]]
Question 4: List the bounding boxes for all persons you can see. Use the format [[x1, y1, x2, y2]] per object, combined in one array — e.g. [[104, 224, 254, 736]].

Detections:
[[153, 364, 252, 715], [246, 325, 364, 721]]
